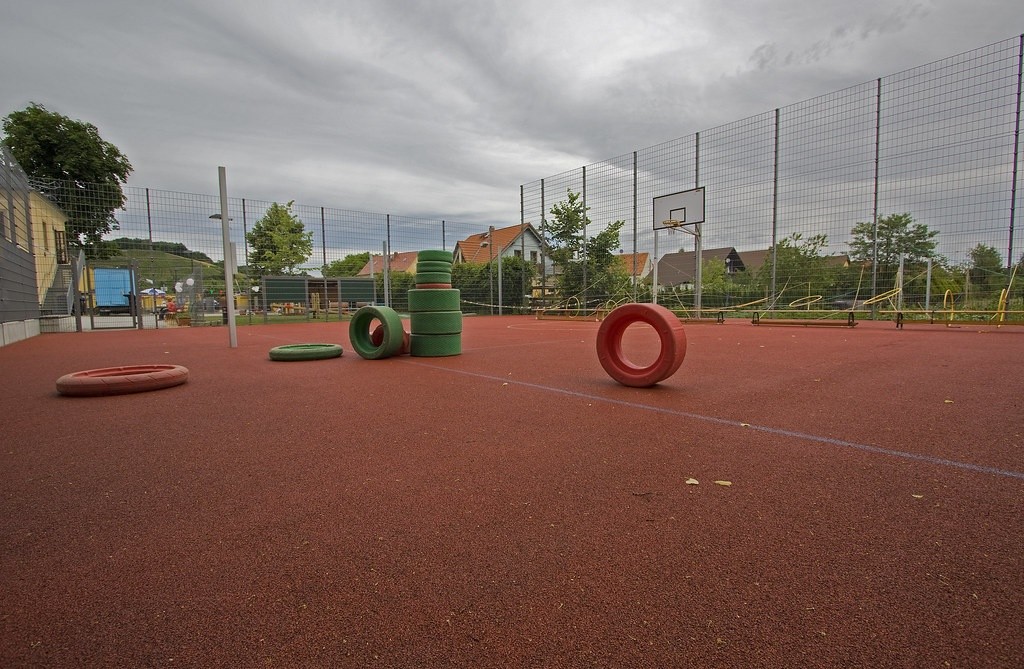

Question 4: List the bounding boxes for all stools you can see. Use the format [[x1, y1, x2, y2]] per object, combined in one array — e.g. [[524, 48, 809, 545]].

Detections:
[[168, 312, 176, 325]]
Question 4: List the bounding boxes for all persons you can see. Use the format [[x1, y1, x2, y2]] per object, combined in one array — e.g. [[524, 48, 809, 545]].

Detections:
[[157, 296, 177, 321]]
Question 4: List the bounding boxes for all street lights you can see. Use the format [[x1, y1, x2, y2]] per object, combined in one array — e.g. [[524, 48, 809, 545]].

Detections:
[[480, 226, 494, 316]]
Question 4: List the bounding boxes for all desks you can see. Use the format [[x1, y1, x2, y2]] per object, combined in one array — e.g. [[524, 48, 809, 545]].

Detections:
[[152, 306, 188, 319]]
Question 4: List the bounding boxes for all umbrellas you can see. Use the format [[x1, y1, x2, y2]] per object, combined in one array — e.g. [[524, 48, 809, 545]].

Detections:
[[140, 288, 166, 312]]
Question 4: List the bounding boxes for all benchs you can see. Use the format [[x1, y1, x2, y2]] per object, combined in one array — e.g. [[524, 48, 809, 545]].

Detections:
[[151, 312, 176, 316], [329, 300, 349, 313]]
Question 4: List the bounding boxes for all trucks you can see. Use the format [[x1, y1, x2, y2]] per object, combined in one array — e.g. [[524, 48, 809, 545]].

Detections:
[[93, 267, 138, 317]]
